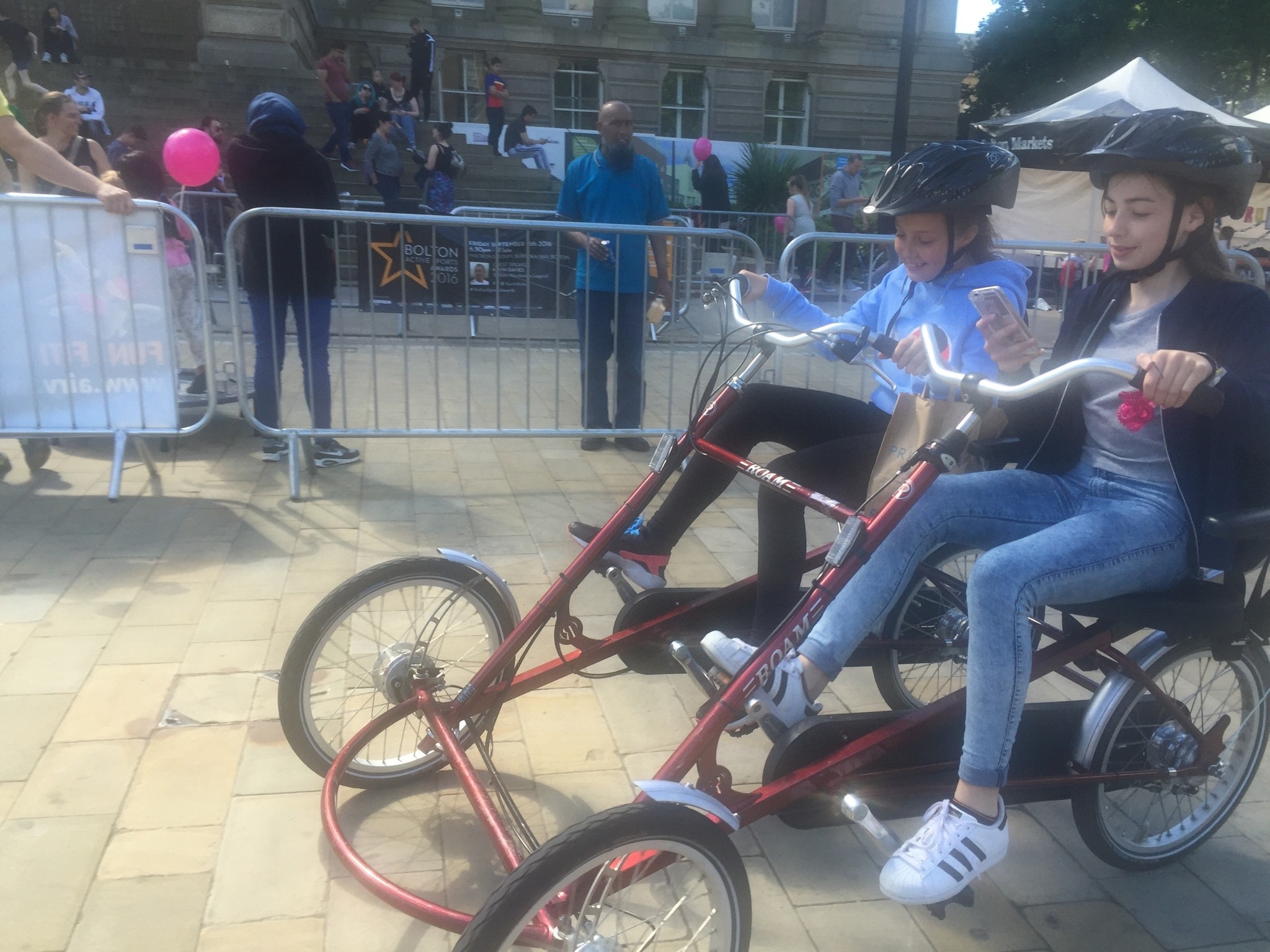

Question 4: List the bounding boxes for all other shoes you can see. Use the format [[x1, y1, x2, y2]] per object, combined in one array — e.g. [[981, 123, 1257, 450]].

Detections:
[[843, 278, 861, 291], [341, 158, 358, 171], [550, 174, 562, 181], [186, 371, 207, 394], [814, 278, 836, 292], [59, 52, 69, 63], [42, 51, 52, 63], [321, 150, 339, 160], [580, 437, 607, 450], [347, 142, 356, 151], [614, 437, 650, 452]]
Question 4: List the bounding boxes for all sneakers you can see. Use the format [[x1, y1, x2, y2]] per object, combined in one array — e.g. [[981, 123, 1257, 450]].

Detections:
[[567, 513, 671, 590], [312, 439, 360, 468], [699, 629, 823, 736], [261, 436, 289, 462], [695, 687, 756, 731], [879, 790, 1010, 905]]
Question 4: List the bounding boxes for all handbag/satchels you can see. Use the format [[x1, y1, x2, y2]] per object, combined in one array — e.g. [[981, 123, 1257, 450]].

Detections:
[[450, 148, 465, 179]]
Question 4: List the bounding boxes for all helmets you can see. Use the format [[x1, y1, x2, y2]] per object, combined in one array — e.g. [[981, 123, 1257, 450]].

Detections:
[[1075, 107, 1261, 220], [862, 140, 1020, 216]]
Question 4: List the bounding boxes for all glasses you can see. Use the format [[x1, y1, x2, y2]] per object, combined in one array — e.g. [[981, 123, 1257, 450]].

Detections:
[[362, 86, 371, 92]]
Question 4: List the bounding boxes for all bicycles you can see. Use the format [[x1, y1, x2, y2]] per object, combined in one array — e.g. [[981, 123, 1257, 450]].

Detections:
[[274, 276, 1269, 952]]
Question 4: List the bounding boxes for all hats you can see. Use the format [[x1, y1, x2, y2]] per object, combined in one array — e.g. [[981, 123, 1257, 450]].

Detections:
[[73, 69, 91, 79]]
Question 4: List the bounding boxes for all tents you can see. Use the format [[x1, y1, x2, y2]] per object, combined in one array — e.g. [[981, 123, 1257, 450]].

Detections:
[[968, 56, 1270, 267]]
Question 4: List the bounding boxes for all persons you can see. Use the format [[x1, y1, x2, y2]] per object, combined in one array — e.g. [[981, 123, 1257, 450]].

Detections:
[[569, 139, 1035, 693], [1058, 239, 1088, 313], [691, 155, 732, 251], [0, 0, 557, 472], [824, 155, 869, 280], [1217, 227, 1237, 274], [558, 101, 672, 452], [696, 108, 1270, 908], [784, 175, 815, 289]]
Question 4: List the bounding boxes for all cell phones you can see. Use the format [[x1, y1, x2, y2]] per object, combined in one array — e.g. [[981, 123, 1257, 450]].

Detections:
[[969, 285, 1040, 357]]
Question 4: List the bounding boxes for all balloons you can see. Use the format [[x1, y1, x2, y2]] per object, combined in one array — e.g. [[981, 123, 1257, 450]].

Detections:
[[774, 215, 789, 234], [692, 137, 713, 161], [160, 127, 222, 187]]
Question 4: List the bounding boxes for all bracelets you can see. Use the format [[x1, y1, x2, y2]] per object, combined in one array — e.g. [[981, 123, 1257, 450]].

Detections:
[[1194, 351, 1216, 383]]
